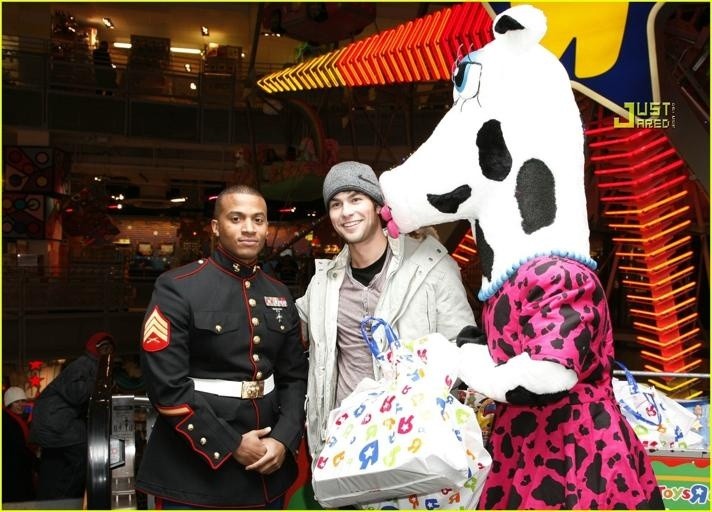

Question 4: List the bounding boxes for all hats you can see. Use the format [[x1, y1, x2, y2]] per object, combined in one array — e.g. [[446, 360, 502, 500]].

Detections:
[[4, 387, 27, 408], [322, 161, 385, 210]]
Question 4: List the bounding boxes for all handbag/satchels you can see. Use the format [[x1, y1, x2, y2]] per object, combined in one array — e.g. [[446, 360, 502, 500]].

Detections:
[[310, 317, 493, 509], [612, 361, 703, 457]]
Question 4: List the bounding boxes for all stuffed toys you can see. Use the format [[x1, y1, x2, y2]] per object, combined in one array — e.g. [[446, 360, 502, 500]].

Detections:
[[378, 4, 666, 510]]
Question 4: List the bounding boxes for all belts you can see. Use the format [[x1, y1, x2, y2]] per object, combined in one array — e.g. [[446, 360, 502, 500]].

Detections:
[[187, 373, 275, 399]]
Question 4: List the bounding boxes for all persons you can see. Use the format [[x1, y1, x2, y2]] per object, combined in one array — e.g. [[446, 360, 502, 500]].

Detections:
[[295, 161, 481, 459], [134, 185, 309, 511], [3, 331, 115, 503]]
